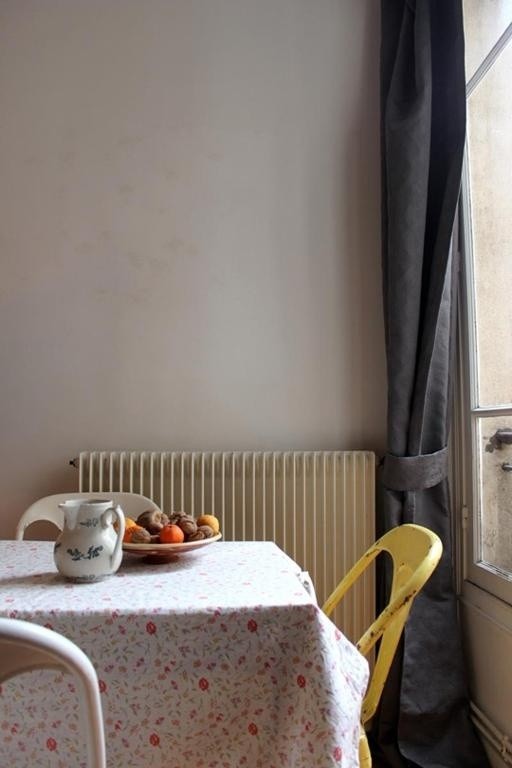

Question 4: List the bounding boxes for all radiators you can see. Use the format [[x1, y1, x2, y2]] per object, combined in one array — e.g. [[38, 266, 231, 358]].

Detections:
[[67, 448, 377, 735]]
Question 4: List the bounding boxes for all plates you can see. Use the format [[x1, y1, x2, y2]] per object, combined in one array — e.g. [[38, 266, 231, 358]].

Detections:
[[120, 533, 223, 557]]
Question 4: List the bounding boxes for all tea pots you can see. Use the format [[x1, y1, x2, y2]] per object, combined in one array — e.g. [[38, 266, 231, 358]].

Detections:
[[52, 499, 126, 584]]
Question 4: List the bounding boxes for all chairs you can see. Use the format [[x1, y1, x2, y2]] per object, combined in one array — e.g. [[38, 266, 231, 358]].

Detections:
[[322, 522, 444, 767], [2, 617, 106, 767], [16, 492, 161, 542]]
